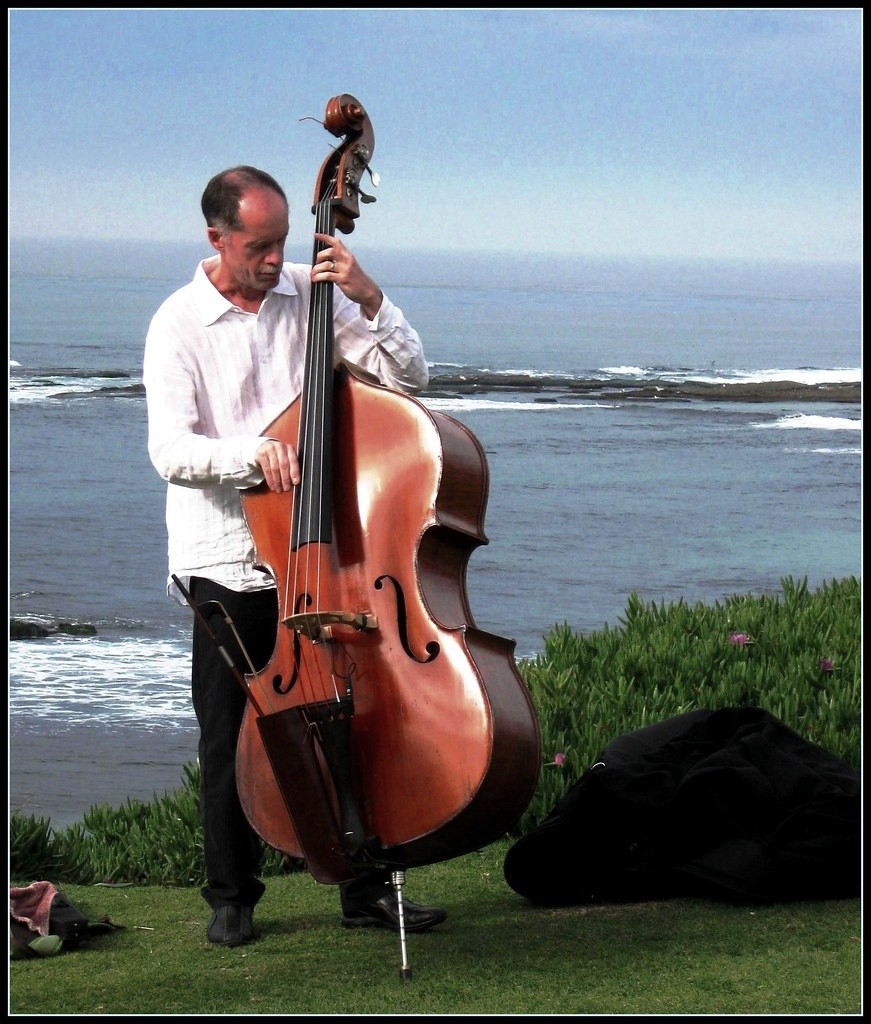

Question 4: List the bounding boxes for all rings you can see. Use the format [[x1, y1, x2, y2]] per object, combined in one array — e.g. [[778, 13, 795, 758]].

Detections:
[[331, 261, 337, 272]]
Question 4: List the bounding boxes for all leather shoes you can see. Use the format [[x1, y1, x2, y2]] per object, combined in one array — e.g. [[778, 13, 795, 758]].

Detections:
[[207, 905, 255, 947], [342, 892, 448, 933]]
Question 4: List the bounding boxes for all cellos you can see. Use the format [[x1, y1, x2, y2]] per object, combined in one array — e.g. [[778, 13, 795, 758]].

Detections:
[[232, 93, 542, 974]]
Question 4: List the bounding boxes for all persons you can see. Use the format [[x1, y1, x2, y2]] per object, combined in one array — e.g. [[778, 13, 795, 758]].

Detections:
[[142, 165, 450, 947]]
